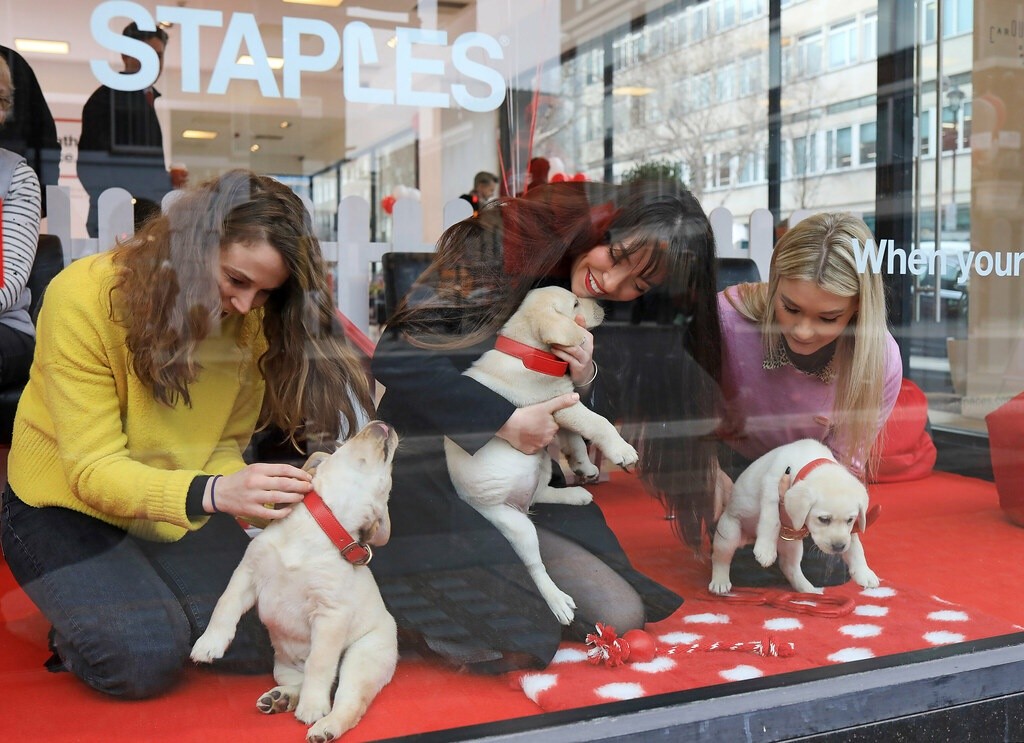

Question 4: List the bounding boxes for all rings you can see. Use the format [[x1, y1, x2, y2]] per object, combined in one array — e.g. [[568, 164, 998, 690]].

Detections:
[[581, 336, 587, 344]]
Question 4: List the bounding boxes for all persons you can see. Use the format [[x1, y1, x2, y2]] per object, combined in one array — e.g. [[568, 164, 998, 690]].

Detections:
[[702, 212, 903, 587], [370, 178, 736, 672], [528, 157, 550, 188], [75, 21, 172, 238], [1, 169, 372, 700], [471, 171, 499, 209], [0, 59, 40, 395]]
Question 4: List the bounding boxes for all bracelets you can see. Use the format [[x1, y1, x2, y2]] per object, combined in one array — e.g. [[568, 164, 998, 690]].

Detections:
[[211, 474, 223, 514], [575, 361, 597, 388]]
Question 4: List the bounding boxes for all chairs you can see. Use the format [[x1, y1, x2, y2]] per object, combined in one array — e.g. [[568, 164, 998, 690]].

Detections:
[[382, 250, 468, 332]]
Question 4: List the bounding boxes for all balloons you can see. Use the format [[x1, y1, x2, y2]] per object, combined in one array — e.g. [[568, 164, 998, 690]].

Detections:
[[381, 185, 421, 214]]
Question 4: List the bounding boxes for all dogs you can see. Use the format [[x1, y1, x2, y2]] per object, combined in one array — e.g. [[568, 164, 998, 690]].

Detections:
[[443, 286, 640, 625], [190, 420, 398, 743], [708, 438, 884, 594]]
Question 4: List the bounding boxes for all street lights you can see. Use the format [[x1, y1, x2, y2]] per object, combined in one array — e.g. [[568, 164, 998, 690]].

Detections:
[[946, 84, 975, 232]]
